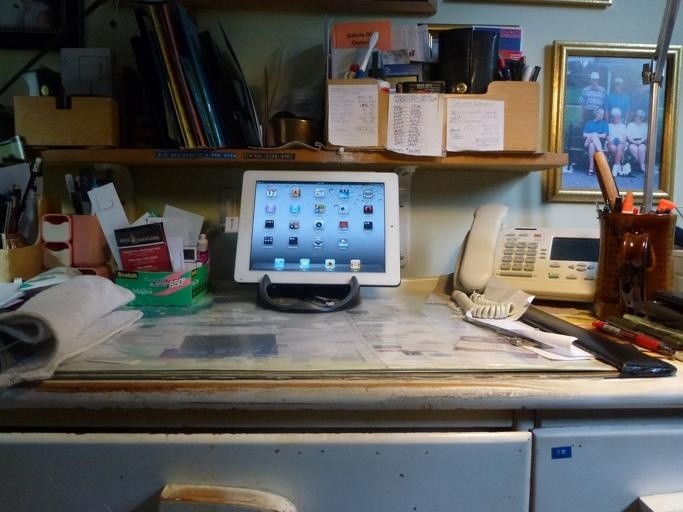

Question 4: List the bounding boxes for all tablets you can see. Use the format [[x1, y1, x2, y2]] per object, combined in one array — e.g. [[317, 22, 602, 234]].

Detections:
[[234, 168, 402, 288]]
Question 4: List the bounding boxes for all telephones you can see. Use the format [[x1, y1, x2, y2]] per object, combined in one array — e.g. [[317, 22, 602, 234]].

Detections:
[[453, 203, 601, 303]]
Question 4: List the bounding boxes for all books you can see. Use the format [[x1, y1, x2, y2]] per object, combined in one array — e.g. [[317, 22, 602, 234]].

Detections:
[[132, 3, 263, 153], [329, 18, 520, 92]]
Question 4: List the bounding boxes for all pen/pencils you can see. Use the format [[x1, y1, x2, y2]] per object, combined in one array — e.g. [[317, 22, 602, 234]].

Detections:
[[495, 53, 541, 82], [0, 157, 114, 250], [591, 299, 683, 357], [596, 192, 677, 217]]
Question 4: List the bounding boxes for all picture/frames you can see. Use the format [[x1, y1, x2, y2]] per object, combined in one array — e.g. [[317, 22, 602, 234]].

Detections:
[[542, 39, 682, 204]]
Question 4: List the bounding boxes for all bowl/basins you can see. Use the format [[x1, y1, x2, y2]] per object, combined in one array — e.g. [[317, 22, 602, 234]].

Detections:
[[273, 115, 322, 147]]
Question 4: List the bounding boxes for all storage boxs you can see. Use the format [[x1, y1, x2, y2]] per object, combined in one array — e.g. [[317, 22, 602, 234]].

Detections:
[[12, 92, 117, 151], [329, 77, 548, 159]]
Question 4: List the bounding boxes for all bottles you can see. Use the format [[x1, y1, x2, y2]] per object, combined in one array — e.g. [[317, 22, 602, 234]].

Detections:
[[197, 234, 208, 266]]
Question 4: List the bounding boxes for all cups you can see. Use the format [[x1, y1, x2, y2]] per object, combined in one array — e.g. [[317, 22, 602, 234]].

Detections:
[[592, 212, 678, 324]]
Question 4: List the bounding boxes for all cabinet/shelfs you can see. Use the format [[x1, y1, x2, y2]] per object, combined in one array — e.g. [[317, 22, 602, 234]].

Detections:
[[0, 274, 682, 511]]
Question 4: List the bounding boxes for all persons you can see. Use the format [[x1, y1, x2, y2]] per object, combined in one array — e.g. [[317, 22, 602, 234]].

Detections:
[[578, 69, 650, 177]]
